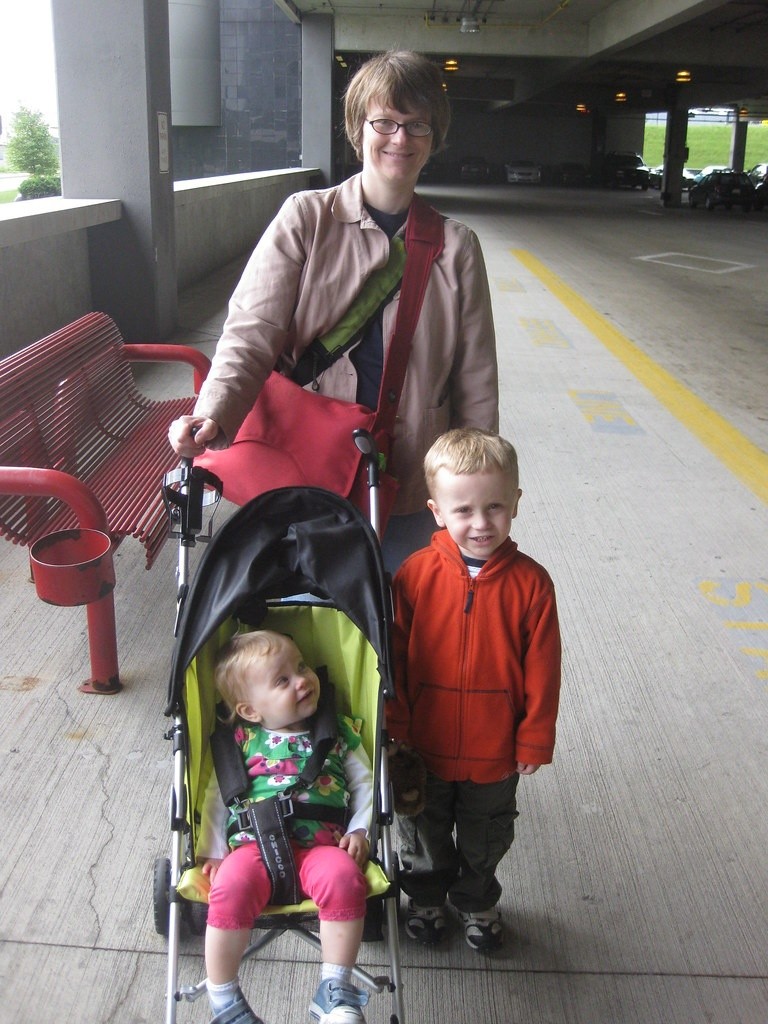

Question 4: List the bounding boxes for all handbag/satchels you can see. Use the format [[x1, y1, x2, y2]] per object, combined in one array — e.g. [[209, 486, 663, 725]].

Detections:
[[191, 369, 402, 536]]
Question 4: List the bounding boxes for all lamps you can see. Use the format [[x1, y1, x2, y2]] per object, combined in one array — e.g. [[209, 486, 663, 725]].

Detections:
[[739, 100, 747, 116], [459, 0, 480, 33], [615, 87, 627, 101], [443, 56, 460, 71], [675, 67, 691, 82], [576, 100, 586, 112]]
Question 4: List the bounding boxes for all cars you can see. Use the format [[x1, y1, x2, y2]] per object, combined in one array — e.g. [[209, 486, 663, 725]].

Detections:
[[688, 169, 756, 213], [503, 157, 543, 185], [648, 163, 702, 189], [749, 165, 768, 208]]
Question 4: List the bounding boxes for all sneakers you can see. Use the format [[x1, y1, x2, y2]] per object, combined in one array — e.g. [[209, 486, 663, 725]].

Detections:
[[460, 910, 508, 950], [204, 988, 261, 1024], [405, 895, 447, 942], [310, 979, 372, 1024]]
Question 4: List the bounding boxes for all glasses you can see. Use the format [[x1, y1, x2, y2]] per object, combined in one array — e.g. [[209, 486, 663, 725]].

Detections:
[[363, 117, 436, 139]]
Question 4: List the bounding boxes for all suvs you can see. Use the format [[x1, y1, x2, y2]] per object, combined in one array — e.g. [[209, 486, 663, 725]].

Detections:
[[596, 148, 651, 191]]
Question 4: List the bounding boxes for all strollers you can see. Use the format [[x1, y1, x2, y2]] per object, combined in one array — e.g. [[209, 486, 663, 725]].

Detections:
[[153, 425, 408, 1024]]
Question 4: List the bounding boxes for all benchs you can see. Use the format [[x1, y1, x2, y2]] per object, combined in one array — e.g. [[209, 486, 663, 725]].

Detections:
[[0, 311, 211, 694]]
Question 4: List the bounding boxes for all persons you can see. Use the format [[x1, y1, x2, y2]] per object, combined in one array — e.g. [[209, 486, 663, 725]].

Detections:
[[168, 51, 501, 587], [387, 430, 561, 950], [197, 629, 377, 1024]]
